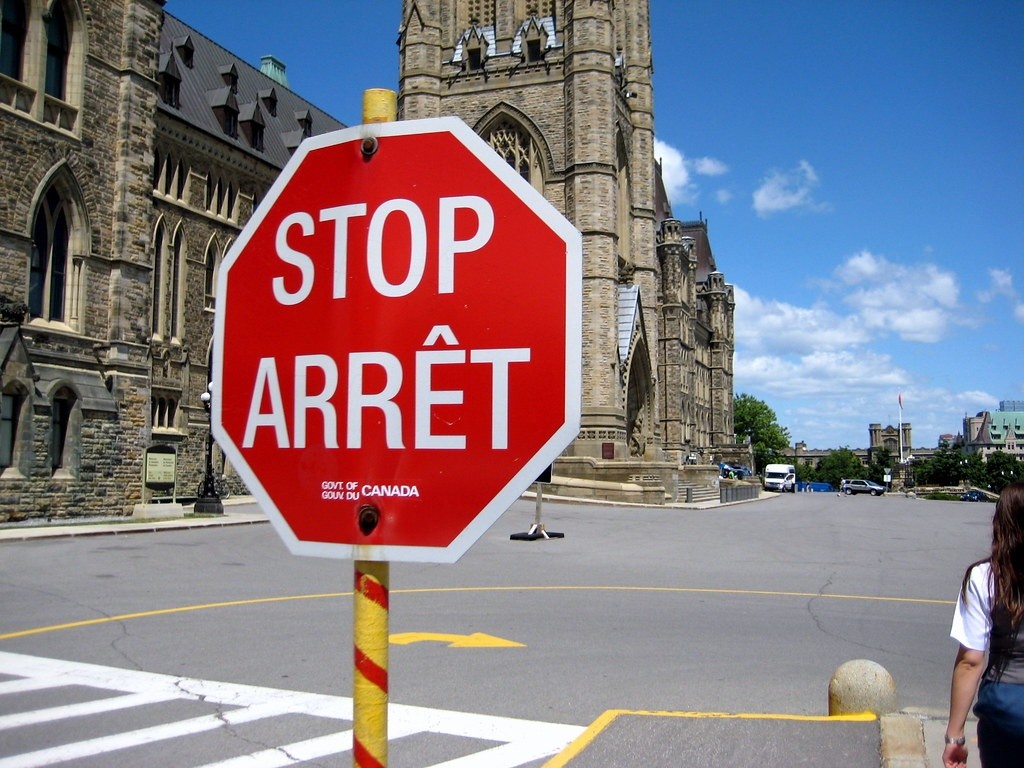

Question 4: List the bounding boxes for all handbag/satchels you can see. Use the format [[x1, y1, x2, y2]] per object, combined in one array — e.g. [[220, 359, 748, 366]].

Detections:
[[973, 682, 1024, 738]]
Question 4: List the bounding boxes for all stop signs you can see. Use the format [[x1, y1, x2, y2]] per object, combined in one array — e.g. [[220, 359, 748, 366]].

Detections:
[[205, 115, 582, 567]]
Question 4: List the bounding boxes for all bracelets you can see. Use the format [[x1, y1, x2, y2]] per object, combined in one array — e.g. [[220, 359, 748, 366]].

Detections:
[[945, 736, 965, 745]]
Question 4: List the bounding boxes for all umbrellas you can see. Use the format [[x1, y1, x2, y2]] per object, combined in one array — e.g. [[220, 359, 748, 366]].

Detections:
[[720, 464, 731, 469]]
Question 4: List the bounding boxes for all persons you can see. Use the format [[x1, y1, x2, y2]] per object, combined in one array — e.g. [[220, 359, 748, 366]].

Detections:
[[805, 479, 810, 488], [791, 476, 795, 493], [943, 483, 1024, 768], [838, 477, 847, 497], [724, 464, 734, 479]]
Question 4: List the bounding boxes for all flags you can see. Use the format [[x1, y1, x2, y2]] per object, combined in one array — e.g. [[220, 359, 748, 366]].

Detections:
[[898, 394, 904, 409]]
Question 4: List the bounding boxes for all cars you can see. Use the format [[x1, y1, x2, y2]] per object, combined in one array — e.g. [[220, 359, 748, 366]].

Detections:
[[959, 490, 986, 502]]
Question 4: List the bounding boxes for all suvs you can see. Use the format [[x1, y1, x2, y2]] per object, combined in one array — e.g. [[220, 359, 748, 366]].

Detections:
[[843, 479, 887, 496]]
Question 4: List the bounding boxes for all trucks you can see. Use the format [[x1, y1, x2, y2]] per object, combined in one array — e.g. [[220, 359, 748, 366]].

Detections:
[[763, 463, 796, 493]]
